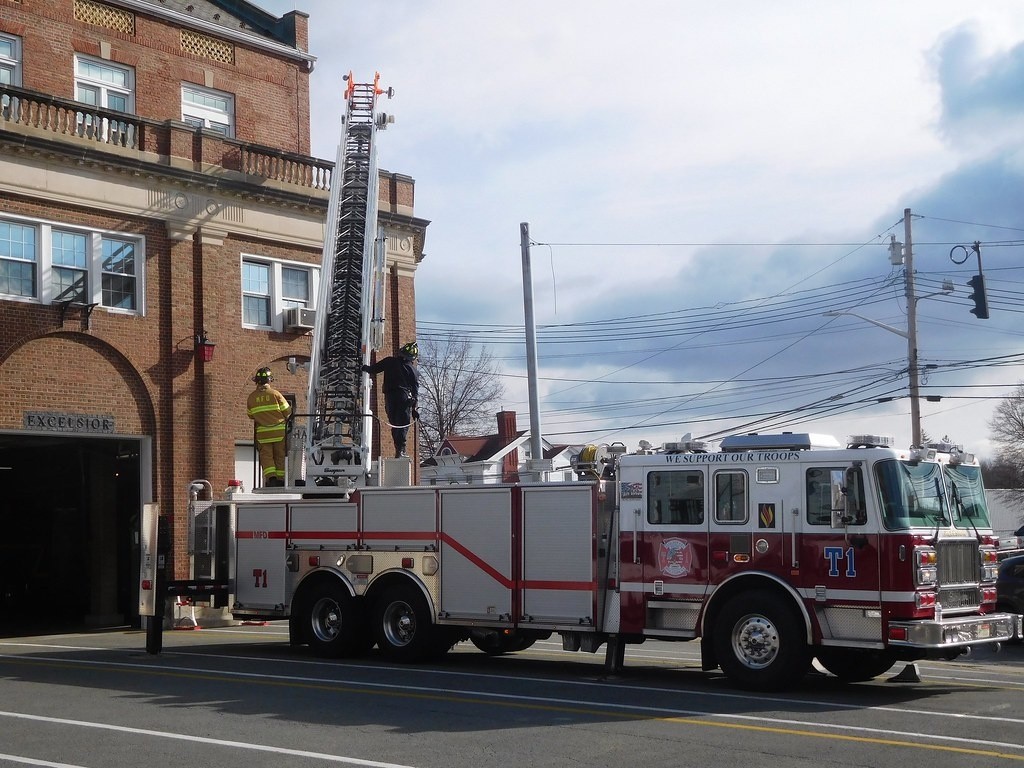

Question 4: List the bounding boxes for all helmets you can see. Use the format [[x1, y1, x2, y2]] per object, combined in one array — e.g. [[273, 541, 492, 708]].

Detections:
[[254, 366, 274, 385], [398, 341, 419, 362]]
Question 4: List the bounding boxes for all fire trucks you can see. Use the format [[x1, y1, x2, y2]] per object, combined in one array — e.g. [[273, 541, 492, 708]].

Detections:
[[146, 70, 1024, 697]]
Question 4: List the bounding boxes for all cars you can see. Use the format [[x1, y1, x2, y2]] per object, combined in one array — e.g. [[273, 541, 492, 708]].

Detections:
[[997, 555, 1024, 648]]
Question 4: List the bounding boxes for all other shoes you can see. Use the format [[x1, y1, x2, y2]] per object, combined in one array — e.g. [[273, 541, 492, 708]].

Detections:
[[263, 476, 284, 487]]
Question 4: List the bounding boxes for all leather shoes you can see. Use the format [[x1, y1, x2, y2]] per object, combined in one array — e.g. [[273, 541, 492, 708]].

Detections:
[[395, 452, 410, 459]]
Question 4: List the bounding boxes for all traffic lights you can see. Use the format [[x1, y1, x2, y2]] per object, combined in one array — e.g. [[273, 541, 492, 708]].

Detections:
[[966, 275, 990, 320]]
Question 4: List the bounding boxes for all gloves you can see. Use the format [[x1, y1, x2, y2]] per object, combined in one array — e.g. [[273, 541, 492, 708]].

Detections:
[[361, 364, 367, 372], [411, 410, 420, 422]]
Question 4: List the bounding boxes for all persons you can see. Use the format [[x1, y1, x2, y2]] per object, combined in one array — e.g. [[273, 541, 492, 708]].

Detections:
[[247, 367, 292, 487], [360, 343, 419, 458]]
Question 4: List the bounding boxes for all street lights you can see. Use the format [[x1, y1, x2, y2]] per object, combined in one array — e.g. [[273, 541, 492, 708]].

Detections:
[[823, 312, 922, 451]]
